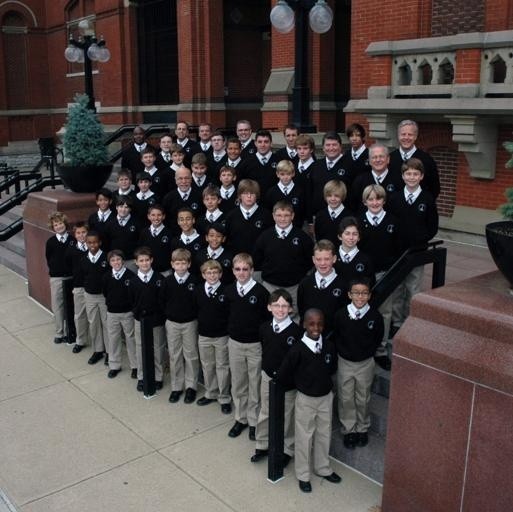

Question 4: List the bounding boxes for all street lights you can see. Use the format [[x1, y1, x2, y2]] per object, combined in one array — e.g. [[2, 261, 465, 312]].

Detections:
[[63, 19, 110, 114], [270, 0, 333, 134]]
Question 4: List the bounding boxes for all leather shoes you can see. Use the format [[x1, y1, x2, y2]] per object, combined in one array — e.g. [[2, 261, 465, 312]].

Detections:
[[88, 352, 102, 365], [55, 337, 63, 344], [358, 431, 368, 446], [169, 390, 182, 402], [387, 323, 398, 342], [104, 354, 109, 365], [251, 449, 269, 462], [72, 344, 84, 353], [221, 404, 231, 414], [184, 389, 196, 403], [197, 396, 215, 405], [318, 471, 341, 483], [249, 427, 256, 440], [345, 433, 355, 448], [376, 354, 393, 371], [137, 380, 143, 391], [108, 368, 122, 378], [284, 452, 290, 467], [228, 419, 248, 437], [131, 368, 138, 379], [155, 381, 163, 389], [299, 480, 311, 492]]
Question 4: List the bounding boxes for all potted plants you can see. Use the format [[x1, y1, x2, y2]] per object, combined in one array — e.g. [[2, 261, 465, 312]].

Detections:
[[484, 139, 513, 289], [54, 92, 114, 193]]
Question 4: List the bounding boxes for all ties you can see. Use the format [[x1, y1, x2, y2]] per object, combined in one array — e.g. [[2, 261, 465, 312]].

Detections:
[[153, 230, 157, 237], [331, 212, 335, 221], [261, 157, 267, 166], [225, 191, 229, 200], [215, 156, 219, 161], [119, 218, 124, 226], [178, 280, 183, 285], [281, 230, 285, 240], [203, 144, 207, 151], [209, 252, 215, 259], [239, 287, 244, 297], [354, 312, 361, 320], [241, 143, 245, 150], [59, 237, 64, 243], [283, 186, 290, 195], [328, 162, 334, 171], [403, 152, 407, 161], [93, 258, 96, 263], [316, 344, 321, 354], [144, 276, 148, 283], [407, 194, 413, 205], [376, 176, 381, 185], [275, 324, 280, 333], [320, 279, 326, 290], [291, 152, 294, 159], [208, 288, 212, 297], [80, 244, 86, 251], [140, 194, 145, 200], [182, 194, 188, 200], [372, 217, 378, 227], [138, 147, 143, 152], [197, 178, 201, 187], [100, 213, 105, 223], [344, 254, 350, 264], [246, 212, 250, 220], [179, 141, 183, 147], [353, 152, 357, 160], [209, 214, 213, 222], [165, 155, 170, 163], [231, 163, 234, 168], [116, 274, 119, 279], [185, 238, 190, 245], [300, 166, 305, 173]]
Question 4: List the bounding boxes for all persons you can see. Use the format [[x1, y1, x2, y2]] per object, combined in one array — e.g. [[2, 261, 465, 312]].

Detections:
[[82, 232, 111, 366], [166, 167, 203, 233], [87, 189, 118, 246], [170, 208, 205, 272], [275, 123, 303, 168], [342, 123, 370, 186], [72, 225, 90, 353], [197, 260, 232, 414], [251, 289, 304, 468], [226, 179, 267, 272], [308, 133, 362, 202], [336, 217, 376, 310], [357, 185, 401, 372], [387, 120, 439, 205], [159, 249, 201, 404], [154, 134, 179, 175], [174, 122, 201, 169], [46, 212, 75, 345], [122, 128, 158, 182], [190, 152, 215, 202], [139, 205, 176, 276], [128, 250, 166, 393], [105, 195, 142, 274], [235, 120, 257, 158], [297, 240, 350, 375], [258, 201, 312, 326], [392, 159, 439, 335], [337, 282, 384, 449], [135, 149, 165, 205], [101, 250, 138, 379], [130, 173, 162, 234], [291, 135, 317, 200], [113, 168, 139, 220], [217, 167, 241, 219], [188, 123, 214, 165], [223, 254, 273, 441], [314, 180, 356, 258], [242, 130, 280, 194], [353, 143, 402, 217], [194, 186, 229, 244], [199, 223, 233, 290], [208, 133, 229, 174], [266, 161, 309, 231], [221, 139, 246, 186], [293, 308, 342, 492]]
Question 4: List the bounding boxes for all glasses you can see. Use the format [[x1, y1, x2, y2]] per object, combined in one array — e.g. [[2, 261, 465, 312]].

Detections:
[[351, 291, 371, 296]]
